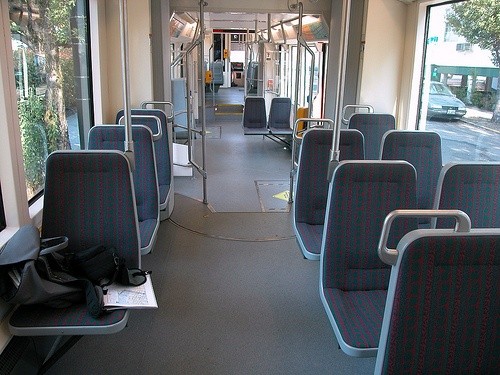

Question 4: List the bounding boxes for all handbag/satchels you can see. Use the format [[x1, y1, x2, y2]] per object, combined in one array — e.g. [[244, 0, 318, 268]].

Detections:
[[72, 243, 120, 284]]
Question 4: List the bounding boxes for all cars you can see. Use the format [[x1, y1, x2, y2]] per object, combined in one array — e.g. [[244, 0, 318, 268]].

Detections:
[[426, 81, 467, 121]]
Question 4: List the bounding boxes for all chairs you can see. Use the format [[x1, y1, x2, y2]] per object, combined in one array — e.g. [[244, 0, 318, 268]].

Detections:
[[203, 62, 224, 93], [379, 130, 442, 229], [242, 96, 293, 147], [374, 210, 500, 375], [86, 124, 160, 255], [116, 108, 174, 210], [319, 160, 418, 357], [430, 161, 500, 229], [8, 150, 141, 375], [171, 78, 196, 140], [347, 113, 396, 160], [247, 62, 257, 93], [294, 128, 366, 261]]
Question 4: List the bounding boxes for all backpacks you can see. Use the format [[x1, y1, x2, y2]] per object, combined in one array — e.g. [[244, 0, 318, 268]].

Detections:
[[0, 225, 105, 318]]
